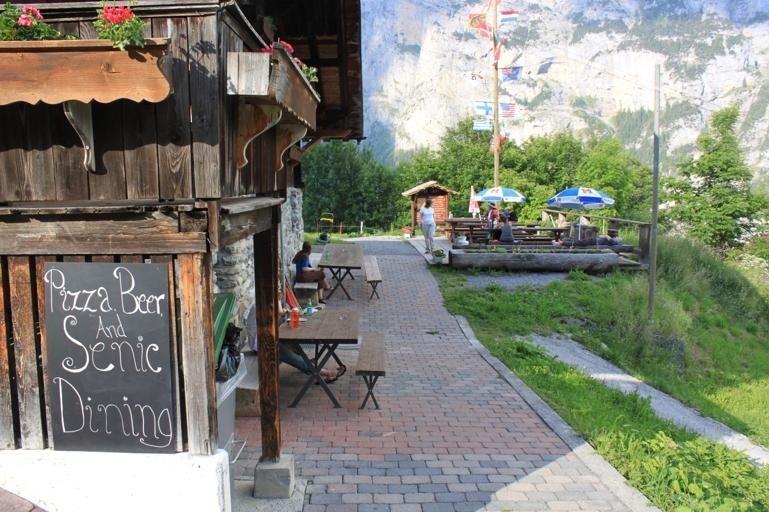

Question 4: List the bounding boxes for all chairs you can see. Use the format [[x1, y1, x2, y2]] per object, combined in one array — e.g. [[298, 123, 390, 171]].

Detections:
[[317, 211, 334, 235]]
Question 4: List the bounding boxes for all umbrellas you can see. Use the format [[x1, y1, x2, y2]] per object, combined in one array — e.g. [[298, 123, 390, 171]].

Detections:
[[547, 186, 616, 241], [468, 185, 480, 218], [474, 186, 528, 210]]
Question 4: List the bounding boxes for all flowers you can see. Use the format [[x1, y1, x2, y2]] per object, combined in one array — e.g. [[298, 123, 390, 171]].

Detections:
[[0, 0, 148, 55], [263, 39, 321, 86]]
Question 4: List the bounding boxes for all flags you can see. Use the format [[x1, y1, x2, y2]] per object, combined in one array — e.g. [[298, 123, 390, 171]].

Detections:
[[468, 0, 525, 133]]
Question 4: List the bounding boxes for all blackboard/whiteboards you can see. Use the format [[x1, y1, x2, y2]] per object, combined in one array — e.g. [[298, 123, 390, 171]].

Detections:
[[42, 262, 177, 453]]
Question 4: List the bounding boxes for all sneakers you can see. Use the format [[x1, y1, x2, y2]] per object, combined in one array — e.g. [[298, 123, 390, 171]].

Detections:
[[319, 369, 336, 380]]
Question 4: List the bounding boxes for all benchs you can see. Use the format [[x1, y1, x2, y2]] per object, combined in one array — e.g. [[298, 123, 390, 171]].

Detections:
[[361, 254, 383, 303], [234, 339, 262, 418], [353, 330, 387, 411]]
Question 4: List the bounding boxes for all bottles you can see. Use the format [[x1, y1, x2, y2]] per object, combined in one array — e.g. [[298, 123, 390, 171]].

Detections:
[[289, 308, 300, 330], [307, 298, 313, 313], [324, 251, 330, 264]]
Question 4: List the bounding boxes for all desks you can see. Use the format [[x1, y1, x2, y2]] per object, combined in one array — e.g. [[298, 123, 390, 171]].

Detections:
[[273, 241, 364, 414], [444, 215, 569, 244]]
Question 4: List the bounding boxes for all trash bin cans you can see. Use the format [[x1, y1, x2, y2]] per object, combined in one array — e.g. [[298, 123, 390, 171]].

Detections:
[[213, 350, 248, 504]]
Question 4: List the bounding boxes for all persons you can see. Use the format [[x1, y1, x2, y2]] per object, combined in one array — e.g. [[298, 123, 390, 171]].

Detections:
[[484, 202, 519, 243], [551, 234, 624, 248], [419, 194, 434, 230], [292, 242, 336, 304], [247, 284, 346, 384], [419, 200, 437, 254]]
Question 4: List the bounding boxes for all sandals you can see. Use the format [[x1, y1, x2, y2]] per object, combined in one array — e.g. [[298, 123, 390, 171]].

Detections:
[[324, 285, 334, 290], [318, 298, 327, 304]]
